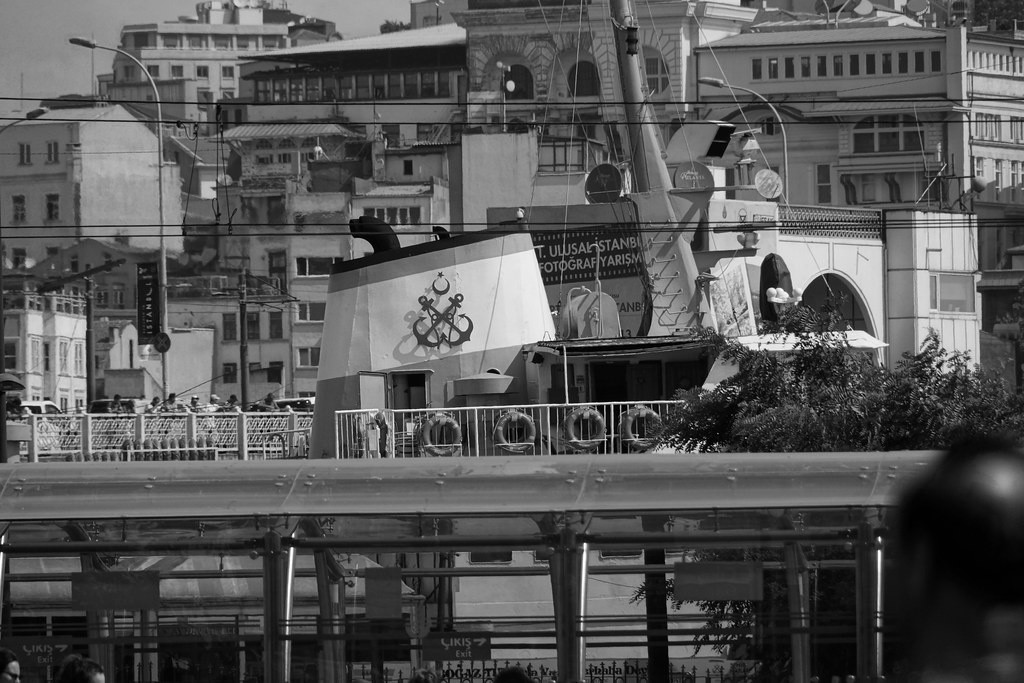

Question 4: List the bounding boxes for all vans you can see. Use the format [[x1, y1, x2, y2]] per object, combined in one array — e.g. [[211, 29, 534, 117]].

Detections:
[[16, 398, 66, 415]]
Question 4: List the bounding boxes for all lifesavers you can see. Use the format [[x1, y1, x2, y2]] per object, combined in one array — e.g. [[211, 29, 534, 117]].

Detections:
[[623, 405, 664, 450], [494, 411, 537, 454], [562, 407, 607, 451], [422, 414, 464, 459]]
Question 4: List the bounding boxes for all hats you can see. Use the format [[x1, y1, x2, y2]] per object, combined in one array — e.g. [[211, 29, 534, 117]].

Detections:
[[210, 394, 220, 399], [191, 395, 199, 399], [168, 393, 176, 397], [268, 393, 275, 397]]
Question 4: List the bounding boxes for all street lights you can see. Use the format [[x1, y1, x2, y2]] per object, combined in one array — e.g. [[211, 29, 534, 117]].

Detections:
[[697, 75, 789, 211], [67, 36, 172, 406]]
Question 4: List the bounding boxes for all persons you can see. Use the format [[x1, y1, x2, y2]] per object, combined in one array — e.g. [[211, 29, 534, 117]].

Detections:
[[107, 392, 280, 413], [5, 398, 29, 421]]
